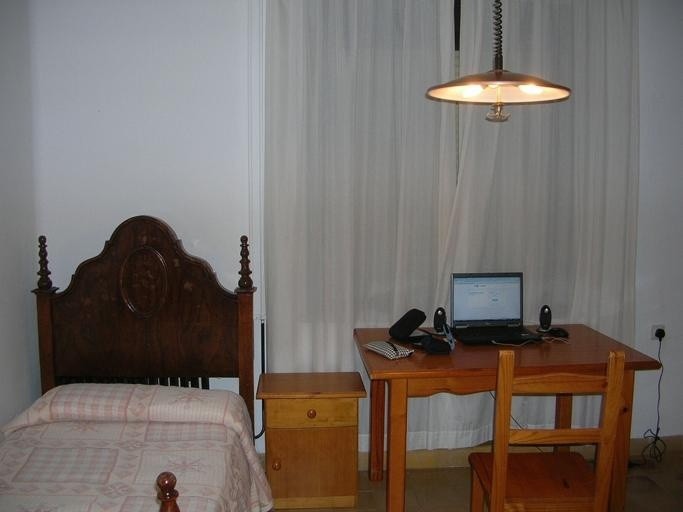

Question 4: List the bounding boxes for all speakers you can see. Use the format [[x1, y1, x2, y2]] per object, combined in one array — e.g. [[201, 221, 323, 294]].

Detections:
[[433, 306, 447, 336], [537, 305, 552, 333]]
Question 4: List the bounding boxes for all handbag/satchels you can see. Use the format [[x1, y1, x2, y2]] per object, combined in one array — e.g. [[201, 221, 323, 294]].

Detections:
[[361, 340, 415, 361]]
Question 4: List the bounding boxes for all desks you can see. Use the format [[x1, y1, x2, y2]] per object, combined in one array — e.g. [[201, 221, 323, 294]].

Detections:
[[353, 323, 662, 512]]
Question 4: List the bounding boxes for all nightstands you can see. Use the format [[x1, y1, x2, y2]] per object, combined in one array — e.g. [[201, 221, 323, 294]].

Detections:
[[255, 372, 367, 511]]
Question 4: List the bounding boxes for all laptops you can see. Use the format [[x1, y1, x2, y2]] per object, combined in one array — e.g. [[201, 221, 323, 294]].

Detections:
[[449, 272, 542, 347]]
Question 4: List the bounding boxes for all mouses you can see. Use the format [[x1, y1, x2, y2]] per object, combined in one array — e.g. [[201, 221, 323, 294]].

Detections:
[[549, 326, 569, 339]]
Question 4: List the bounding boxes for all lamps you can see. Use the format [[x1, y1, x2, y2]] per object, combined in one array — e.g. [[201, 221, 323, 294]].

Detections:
[[425, 0, 572, 121]]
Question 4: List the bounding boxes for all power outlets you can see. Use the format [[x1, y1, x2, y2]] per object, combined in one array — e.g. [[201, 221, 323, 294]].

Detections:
[[650, 324, 666, 343]]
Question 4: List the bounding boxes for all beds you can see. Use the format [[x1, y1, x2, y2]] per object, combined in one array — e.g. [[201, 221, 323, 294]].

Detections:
[[0, 215, 258, 512]]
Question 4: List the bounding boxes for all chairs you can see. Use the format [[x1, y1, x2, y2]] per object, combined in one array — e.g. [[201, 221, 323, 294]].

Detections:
[[468, 348, 625, 512]]
